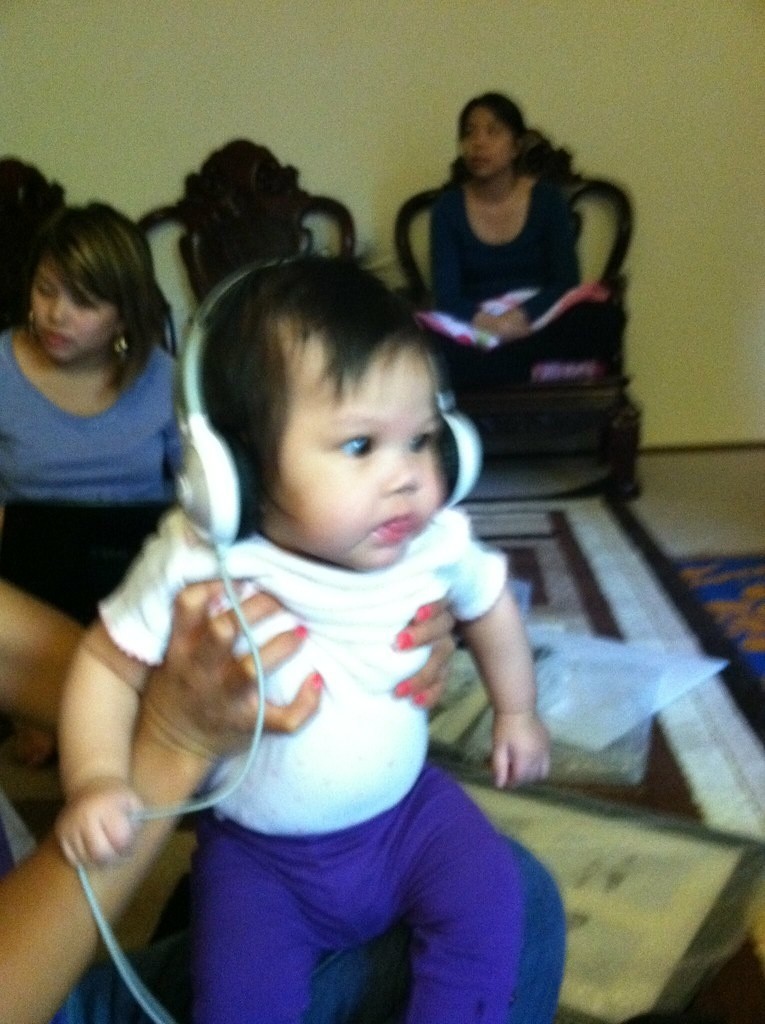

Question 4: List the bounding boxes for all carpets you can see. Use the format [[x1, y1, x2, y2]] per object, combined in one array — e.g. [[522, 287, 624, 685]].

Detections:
[[0, 481, 764, 1023]]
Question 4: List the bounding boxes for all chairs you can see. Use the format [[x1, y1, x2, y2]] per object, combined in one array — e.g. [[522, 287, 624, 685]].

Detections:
[[390, 175, 641, 499], [132, 139, 357, 359]]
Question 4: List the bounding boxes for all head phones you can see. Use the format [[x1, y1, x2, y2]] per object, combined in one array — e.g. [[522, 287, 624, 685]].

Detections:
[[176, 255, 483, 549]]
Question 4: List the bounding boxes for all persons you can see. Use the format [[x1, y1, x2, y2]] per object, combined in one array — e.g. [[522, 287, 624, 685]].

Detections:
[[0, 203, 566, 1023], [423, 92, 624, 392]]
